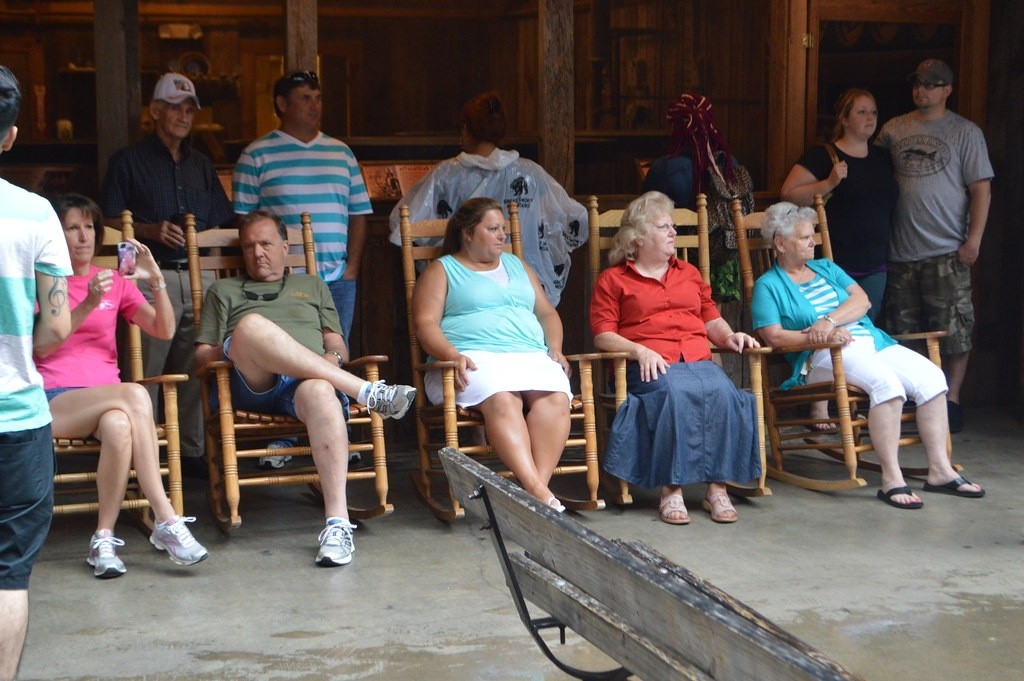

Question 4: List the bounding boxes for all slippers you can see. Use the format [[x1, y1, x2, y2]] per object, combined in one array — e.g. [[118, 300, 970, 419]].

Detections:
[[703, 491, 738, 522], [659, 494, 691, 524], [877, 485, 923, 508], [923, 475, 985, 497]]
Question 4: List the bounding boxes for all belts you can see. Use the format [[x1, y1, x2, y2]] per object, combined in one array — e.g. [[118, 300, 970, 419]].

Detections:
[[154, 260, 190, 271]]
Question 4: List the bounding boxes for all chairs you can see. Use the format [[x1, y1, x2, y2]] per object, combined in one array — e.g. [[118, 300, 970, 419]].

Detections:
[[400, 200, 607, 519], [733, 194, 964, 492], [50, 209, 189, 537], [184, 210, 393, 530], [586, 195, 773, 503]]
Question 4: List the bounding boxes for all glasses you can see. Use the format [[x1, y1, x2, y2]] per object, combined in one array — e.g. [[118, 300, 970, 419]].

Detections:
[[241, 275, 285, 301], [911, 78, 949, 91], [648, 224, 678, 232], [280, 70, 321, 97]]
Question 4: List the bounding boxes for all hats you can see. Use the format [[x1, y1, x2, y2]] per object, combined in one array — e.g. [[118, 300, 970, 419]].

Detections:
[[153, 72, 202, 111], [909, 59, 954, 85]]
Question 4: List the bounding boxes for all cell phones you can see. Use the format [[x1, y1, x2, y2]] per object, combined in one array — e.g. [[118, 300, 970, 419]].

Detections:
[[117, 242, 136, 275]]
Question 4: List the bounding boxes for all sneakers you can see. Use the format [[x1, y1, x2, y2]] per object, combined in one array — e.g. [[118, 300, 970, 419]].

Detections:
[[149, 515, 210, 567], [316, 516, 357, 566], [364, 379, 418, 420], [86, 529, 127, 577]]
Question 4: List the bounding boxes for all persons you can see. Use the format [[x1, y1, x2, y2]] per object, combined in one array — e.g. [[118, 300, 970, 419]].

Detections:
[[192, 210, 417, 566], [779, 90, 898, 435], [412, 196, 573, 514], [31, 191, 209, 579], [589, 191, 761, 524], [751, 202, 986, 509], [100, 72, 237, 478], [0, 66, 74, 681], [641, 93, 755, 365], [231, 70, 374, 470], [389, 92, 589, 454], [874, 60, 994, 434]]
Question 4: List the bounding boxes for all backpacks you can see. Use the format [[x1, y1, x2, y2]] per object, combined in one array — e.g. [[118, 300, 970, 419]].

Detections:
[[686, 150, 756, 265]]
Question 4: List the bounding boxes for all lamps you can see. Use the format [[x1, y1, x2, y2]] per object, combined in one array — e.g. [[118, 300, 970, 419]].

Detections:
[[158, 23, 202, 40]]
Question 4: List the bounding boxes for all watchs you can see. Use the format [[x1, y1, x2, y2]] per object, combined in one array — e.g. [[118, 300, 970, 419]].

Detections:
[[824, 314, 838, 327]]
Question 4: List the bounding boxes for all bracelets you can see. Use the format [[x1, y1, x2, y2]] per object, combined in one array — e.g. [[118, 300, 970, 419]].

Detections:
[[326, 351, 344, 367], [151, 281, 167, 291]]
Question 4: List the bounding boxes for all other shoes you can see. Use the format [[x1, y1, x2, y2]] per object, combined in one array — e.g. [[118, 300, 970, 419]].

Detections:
[[347, 440, 361, 465], [851, 410, 868, 429], [805, 418, 838, 436], [945, 400, 964, 433], [548, 497, 566, 512], [257, 439, 293, 470]]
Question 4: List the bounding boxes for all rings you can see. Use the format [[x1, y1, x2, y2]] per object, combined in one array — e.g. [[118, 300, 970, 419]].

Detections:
[[95, 286, 100, 290], [843, 170, 845, 174], [838, 336, 842, 341]]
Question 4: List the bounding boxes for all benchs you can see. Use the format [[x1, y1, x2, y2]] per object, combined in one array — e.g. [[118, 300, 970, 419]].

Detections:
[[438, 446, 865, 681]]
